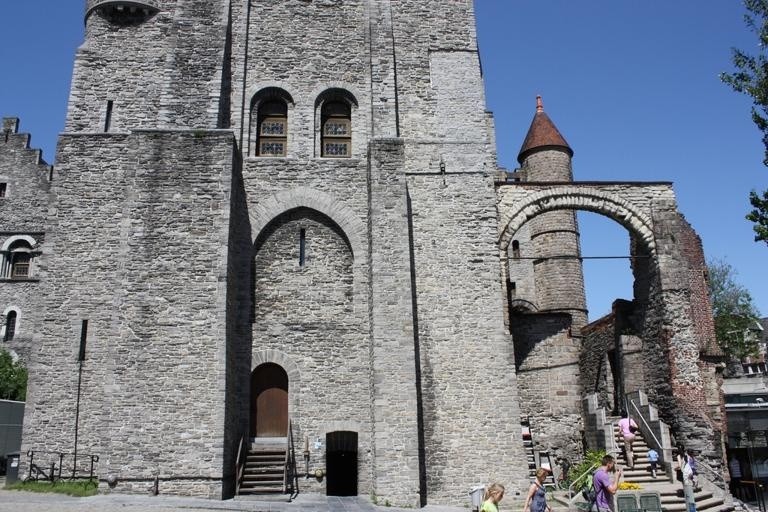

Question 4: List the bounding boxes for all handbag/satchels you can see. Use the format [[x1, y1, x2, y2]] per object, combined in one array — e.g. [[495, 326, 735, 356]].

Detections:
[[629, 416, 638, 434]]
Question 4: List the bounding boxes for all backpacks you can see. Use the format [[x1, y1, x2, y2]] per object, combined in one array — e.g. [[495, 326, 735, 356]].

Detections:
[[581, 468, 606, 505]]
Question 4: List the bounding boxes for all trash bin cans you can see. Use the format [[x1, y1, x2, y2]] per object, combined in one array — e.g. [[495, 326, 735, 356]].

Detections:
[[469, 486, 485, 512], [5, 452, 20, 484], [613, 490, 662, 512]]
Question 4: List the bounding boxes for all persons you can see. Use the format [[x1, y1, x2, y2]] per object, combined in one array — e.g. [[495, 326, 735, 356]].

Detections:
[[686, 449, 698, 487], [647, 446, 659, 478], [674, 443, 693, 488], [683, 475, 698, 511], [523, 468, 553, 512], [728, 454, 747, 501], [557, 458, 570, 490], [480, 483, 505, 512], [618, 411, 638, 467], [591, 455, 622, 512]]
